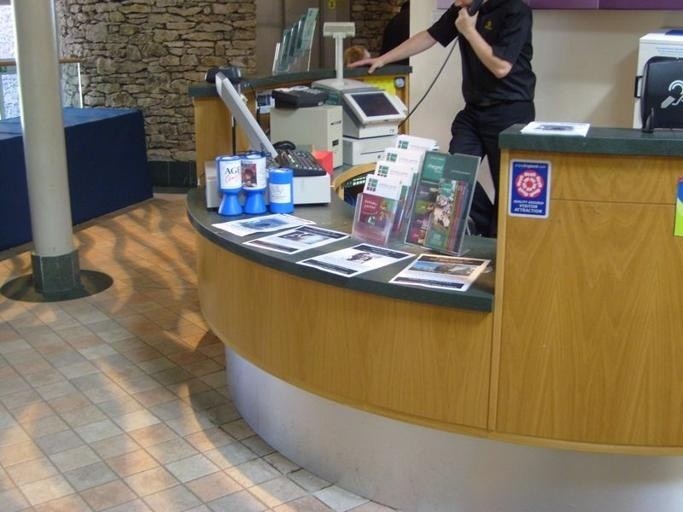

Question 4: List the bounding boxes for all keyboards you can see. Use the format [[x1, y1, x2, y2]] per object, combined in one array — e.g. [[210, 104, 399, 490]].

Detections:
[[281, 150, 326, 176]]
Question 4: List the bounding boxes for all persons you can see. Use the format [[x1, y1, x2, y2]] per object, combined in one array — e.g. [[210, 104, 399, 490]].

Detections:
[[345, 1, 540, 241]]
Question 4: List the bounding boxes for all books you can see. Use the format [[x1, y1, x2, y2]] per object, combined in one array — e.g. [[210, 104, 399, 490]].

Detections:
[[354, 134, 436, 244]]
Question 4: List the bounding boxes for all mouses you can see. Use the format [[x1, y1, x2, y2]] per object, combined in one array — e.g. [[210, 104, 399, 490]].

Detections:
[[272, 141, 295, 150]]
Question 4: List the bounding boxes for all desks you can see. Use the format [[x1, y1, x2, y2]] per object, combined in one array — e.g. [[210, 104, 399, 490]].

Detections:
[[1, 104, 155, 251]]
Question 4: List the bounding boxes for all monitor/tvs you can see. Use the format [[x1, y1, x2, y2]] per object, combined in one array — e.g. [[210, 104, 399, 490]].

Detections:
[[214, 70, 279, 160]]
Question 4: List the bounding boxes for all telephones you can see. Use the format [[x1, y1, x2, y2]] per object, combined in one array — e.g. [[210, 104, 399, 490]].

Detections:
[[457, 1, 482, 41]]
